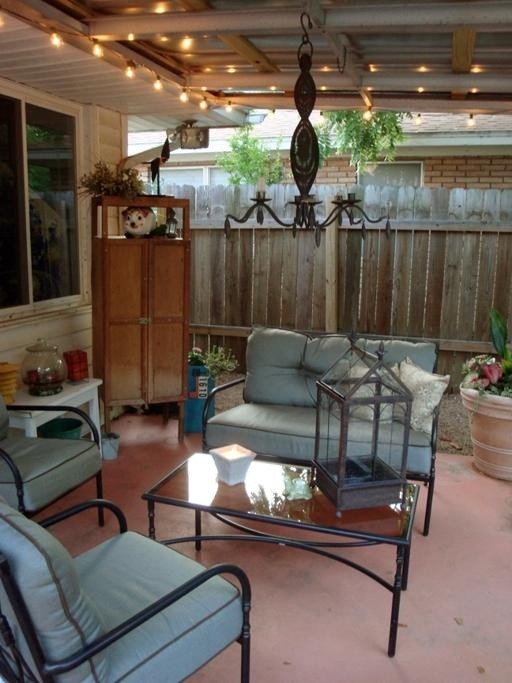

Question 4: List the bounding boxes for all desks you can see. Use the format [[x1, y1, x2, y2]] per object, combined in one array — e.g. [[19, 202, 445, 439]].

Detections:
[[7, 374, 104, 459]]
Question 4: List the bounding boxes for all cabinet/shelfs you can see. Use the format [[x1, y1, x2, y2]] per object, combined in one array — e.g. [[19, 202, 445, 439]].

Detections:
[[89, 192, 192, 445]]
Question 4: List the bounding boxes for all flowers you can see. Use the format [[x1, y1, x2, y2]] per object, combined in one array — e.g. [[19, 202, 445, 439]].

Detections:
[[457, 308, 512, 397]]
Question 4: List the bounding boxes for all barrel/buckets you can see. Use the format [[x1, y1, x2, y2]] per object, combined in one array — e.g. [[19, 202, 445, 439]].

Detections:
[[101, 431, 121, 461], [38, 417, 84, 439]]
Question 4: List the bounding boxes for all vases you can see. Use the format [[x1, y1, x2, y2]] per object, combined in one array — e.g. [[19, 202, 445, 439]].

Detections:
[[460, 382, 512, 485]]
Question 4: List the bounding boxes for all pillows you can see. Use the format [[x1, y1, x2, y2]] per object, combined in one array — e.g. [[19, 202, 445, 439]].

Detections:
[[391, 355, 451, 443], [346, 366, 396, 428]]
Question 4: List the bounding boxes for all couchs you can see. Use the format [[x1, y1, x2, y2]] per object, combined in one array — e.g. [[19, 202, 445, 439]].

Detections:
[[200, 323, 442, 537]]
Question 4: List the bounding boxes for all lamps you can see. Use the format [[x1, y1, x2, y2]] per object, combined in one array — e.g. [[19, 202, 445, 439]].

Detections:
[[227, 98, 476, 129], [51, 26, 212, 112], [218, 12, 395, 250]]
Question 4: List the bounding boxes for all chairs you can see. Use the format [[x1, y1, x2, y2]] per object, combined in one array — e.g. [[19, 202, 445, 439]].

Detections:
[[2, 499, 253, 682], [1, 397, 109, 535]]
